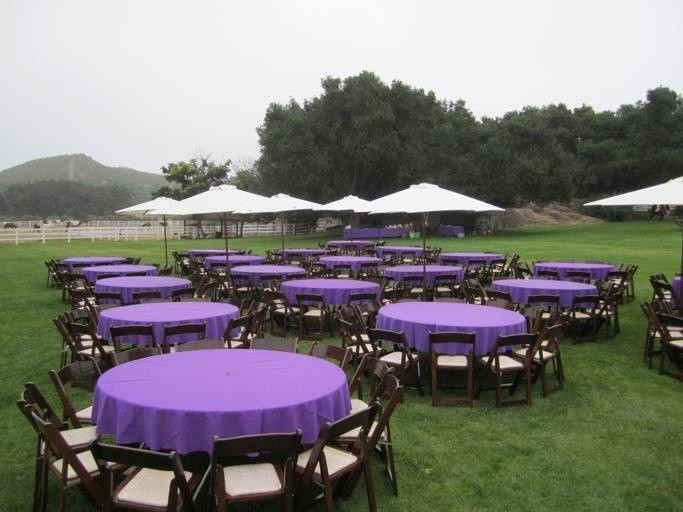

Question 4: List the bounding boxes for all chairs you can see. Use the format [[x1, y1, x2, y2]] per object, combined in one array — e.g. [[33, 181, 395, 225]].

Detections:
[[18, 240, 406, 512], [640, 273, 680, 382], [332, 241, 639, 407]]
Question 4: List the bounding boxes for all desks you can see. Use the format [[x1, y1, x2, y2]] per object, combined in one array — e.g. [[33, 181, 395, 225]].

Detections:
[[437, 223, 463, 239], [382, 226, 413, 238], [342, 226, 380, 242]]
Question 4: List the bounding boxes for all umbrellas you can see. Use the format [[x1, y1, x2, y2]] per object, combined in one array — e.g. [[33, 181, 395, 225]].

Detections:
[[581, 175, 683, 207], [233, 193, 322, 254], [146, 184, 269, 271], [354, 181, 506, 302], [313, 193, 368, 243], [114, 197, 178, 267]]
